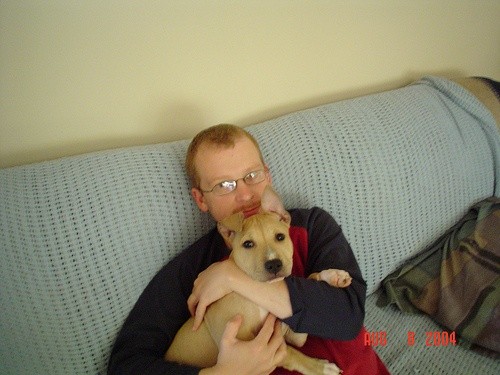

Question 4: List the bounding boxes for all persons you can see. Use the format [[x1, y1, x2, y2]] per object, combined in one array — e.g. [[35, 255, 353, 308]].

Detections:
[[107, 124, 391, 375]]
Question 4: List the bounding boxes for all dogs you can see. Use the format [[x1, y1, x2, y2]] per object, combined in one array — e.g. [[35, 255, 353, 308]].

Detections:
[[164, 184, 353, 375]]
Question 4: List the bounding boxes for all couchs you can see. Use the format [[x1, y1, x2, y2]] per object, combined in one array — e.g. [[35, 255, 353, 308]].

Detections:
[[0, 76, 500, 375]]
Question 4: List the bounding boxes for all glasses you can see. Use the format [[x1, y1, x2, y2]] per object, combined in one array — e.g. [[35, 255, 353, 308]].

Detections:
[[204, 169, 266, 195]]
